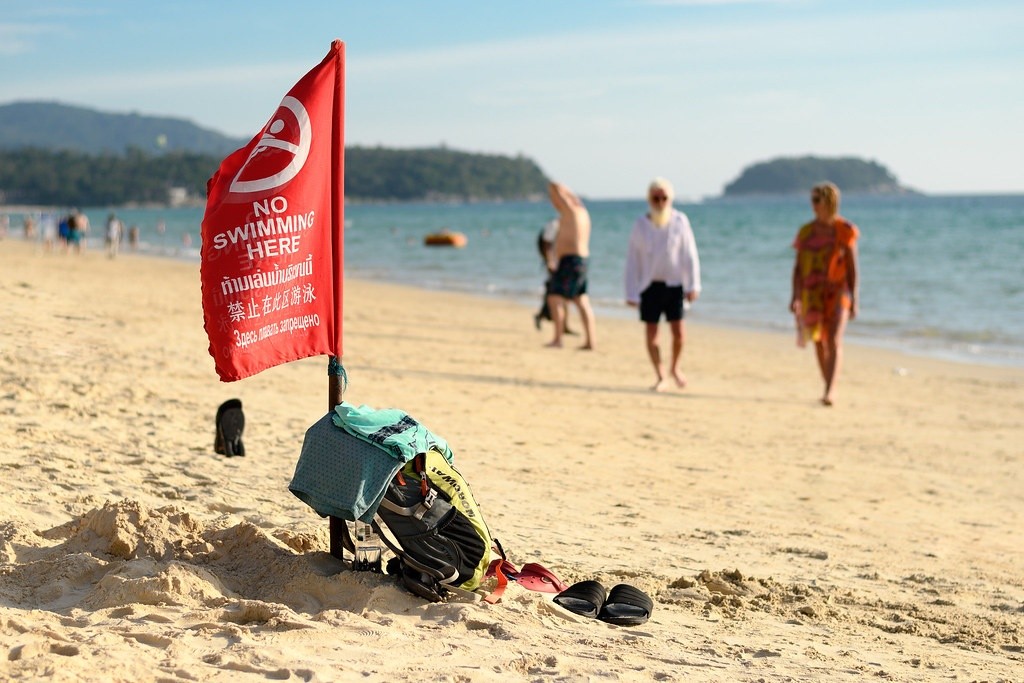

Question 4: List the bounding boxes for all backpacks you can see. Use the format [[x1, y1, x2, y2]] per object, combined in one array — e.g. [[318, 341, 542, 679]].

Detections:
[[369, 448, 493, 604]]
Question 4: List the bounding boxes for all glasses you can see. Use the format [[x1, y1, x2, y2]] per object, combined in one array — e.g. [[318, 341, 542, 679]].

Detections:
[[812, 196, 825, 204], [650, 195, 667, 203]]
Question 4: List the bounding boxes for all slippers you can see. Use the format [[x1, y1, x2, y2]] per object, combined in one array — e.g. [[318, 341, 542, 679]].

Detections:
[[514, 563, 568, 593], [490, 558, 519, 576], [553, 581, 607, 618], [602, 584, 654, 625]]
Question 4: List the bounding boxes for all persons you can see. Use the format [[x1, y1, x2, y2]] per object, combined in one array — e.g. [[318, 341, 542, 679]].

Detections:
[[534, 180, 597, 351], [624, 177, 700, 390], [787, 180, 859, 406], [0, 208, 138, 261]]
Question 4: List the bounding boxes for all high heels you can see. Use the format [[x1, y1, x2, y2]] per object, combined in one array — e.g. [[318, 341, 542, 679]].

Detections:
[[215, 398, 246, 457]]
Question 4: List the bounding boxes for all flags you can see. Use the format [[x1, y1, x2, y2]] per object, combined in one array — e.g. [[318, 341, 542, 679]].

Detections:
[[199, 40, 343, 379]]
